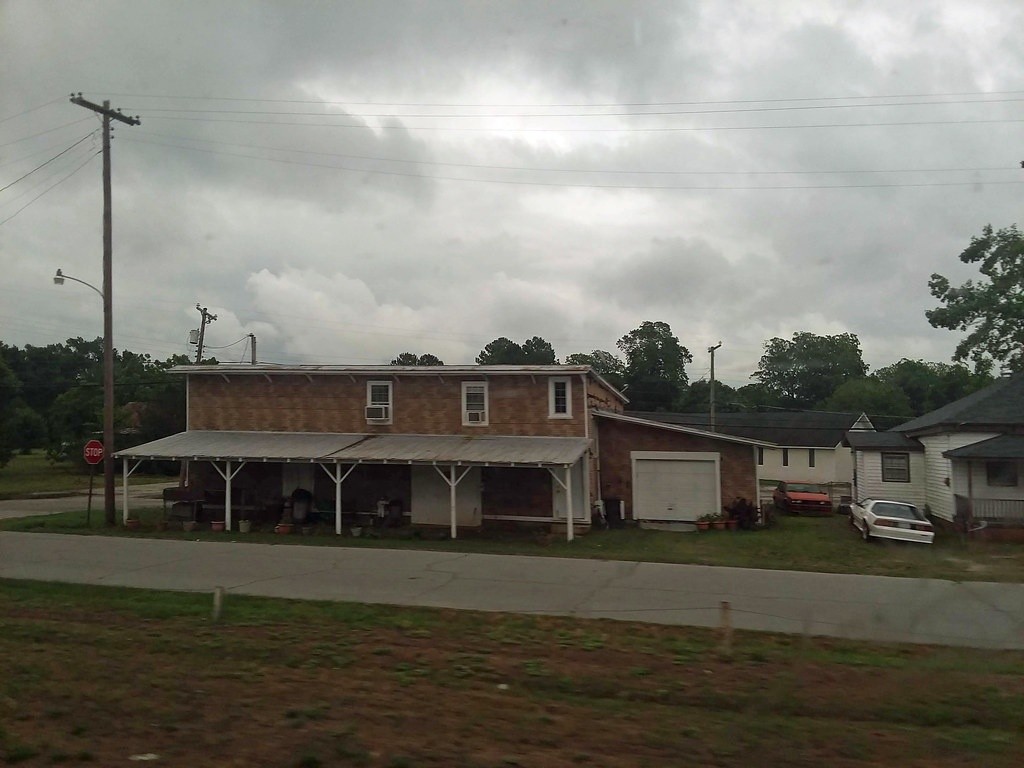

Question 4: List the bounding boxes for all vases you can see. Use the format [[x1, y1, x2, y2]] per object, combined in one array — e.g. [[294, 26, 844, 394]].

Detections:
[[126, 520, 139, 528], [350, 527, 361, 536], [302, 527, 312, 535], [183, 521, 196, 531], [239, 520, 251, 533], [156, 521, 168, 531], [211, 521, 225, 530]]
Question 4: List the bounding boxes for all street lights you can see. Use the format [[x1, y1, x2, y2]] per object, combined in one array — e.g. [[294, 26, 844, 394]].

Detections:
[[52, 268, 118, 529]]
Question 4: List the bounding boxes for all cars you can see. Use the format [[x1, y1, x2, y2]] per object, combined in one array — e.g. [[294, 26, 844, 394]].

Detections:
[[771, 480, 834, 516], [849, 497, 934, 544]]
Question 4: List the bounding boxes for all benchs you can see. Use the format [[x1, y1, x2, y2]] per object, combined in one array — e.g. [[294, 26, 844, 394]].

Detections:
[[202, 490, 255, 520]]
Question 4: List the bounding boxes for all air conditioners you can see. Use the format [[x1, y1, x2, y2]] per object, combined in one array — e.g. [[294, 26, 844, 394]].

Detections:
[[468, 412, 481, 423], [365, 405, 385, 420]]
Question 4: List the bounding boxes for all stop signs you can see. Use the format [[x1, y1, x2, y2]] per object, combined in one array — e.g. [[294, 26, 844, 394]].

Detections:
[[83, 439, 104, 465]]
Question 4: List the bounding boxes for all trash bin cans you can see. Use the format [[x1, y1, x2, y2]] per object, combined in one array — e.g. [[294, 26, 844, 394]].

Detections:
[[291, 488, 312, 521]]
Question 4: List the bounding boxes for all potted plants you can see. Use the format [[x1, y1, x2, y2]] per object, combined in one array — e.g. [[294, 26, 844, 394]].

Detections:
[[695, 497, 759, 534], [277, 517, 295, 535]]
